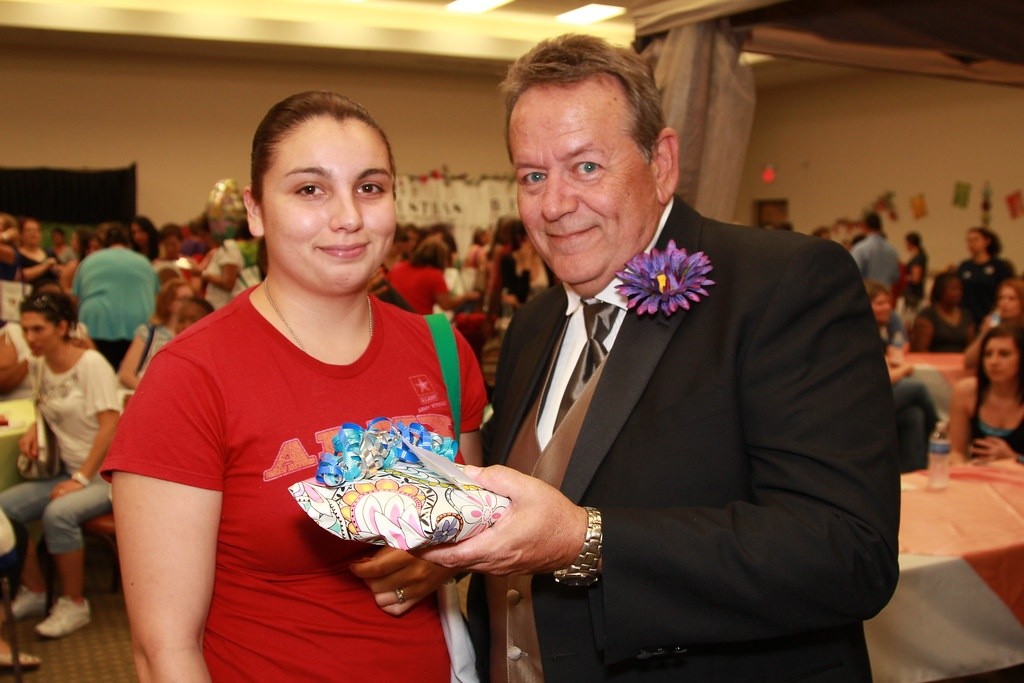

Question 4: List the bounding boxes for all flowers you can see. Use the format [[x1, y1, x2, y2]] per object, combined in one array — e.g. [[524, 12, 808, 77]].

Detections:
[[614, 239, 713, 318]]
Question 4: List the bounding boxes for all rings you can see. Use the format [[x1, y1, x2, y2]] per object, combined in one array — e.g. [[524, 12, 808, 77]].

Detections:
[[58, 489, 63, 494], [396, 588, 406, 603]]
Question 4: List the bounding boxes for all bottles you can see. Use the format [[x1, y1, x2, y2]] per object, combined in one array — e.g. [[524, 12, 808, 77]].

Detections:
[[889, 331, 904, 366], [990, 307, 1003, 329], [927, 422, 950, 489]]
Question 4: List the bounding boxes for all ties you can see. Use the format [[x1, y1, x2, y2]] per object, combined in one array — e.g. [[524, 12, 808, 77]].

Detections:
[[552, 298, 620, 436]]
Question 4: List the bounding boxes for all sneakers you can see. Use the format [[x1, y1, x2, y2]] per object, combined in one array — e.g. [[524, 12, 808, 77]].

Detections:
[[3, 585, 46, 623], [36, 596, 90, 637], [0, 652, 41, 666]]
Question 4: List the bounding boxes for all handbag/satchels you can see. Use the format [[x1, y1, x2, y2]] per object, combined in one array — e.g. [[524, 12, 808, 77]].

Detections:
[[421, 313, 480, 683], [17, 356, 60, 481]]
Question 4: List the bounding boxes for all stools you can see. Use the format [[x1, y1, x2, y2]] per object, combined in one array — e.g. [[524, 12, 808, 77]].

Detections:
[[78, 514, 125, 592]]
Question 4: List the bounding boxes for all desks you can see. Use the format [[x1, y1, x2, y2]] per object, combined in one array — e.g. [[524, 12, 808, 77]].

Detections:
[[861, 455, 1023, 683], [902, 353, 970, 441]]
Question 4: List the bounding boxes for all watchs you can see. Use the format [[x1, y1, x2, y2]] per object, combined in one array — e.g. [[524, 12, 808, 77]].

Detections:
[[551, 505, 603, 588]]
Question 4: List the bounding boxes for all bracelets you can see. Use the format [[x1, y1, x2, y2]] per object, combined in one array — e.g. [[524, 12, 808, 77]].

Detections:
[[72, 472, 90, 487]]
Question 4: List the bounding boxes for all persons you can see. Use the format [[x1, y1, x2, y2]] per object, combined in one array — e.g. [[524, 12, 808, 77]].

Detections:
[[98, 91, 487, 683], [763, 211, 1024, 470], [0, 209, 270, 672], [403, 35, 900, 682], [371, 215, 553, 393]]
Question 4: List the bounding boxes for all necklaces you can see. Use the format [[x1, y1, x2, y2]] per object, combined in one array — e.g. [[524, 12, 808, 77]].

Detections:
[[263, 283, 373, 349]]
